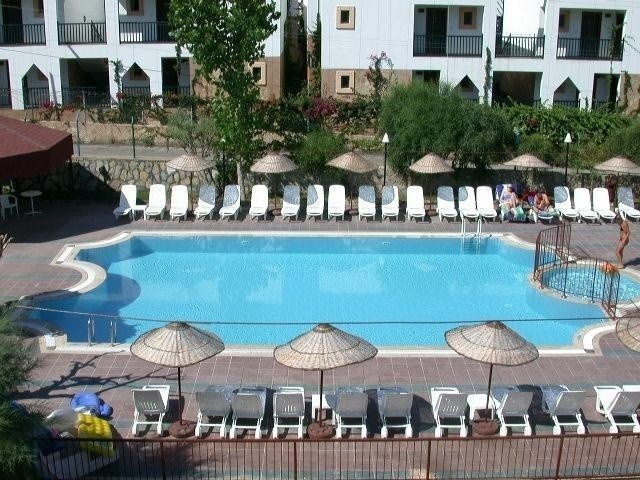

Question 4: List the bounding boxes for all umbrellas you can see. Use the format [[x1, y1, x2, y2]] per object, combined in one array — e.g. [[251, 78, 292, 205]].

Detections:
[[499, 152, 550, 185], [444, 320, 539, 423], [164, 152, 217, 210], [615, 311, 640, 353], [594, 154, 640, 187], [130, 322, 226, 427], [408, 152, 455, 210], [250, 151, 299, 209], [274, 322, 378, 428], [326, 150, 375, 209]]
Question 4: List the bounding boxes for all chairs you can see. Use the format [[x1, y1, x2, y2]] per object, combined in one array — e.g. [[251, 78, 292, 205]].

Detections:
[[541, 385, 586, 436], [250, 186, 269, 221], [574, 187, 597, 223], [376, 386, 414, 438], [131, 385, 171, 438], [329, 186, 345, 221], [490, 387, 533, 437], [0, 194, 18, 221], [406, 186, 425, 221], [553, 186, 579, 224], [476, 186, 497, 222], [146, 184, 167, 220], [437, 186, 457, 223], [194, 186, 216, 220], [169, 186, 189, 221], [456, 186, 479, 222], [529, 185, 554, 223], [592, 187, 616, 224], [382, 186, 400, 221], [112, 185, 137, 221], [194, 385, 305, 440], [306, 186, 324, 220], [592, 385, 639, 434], [358, 186, 376, 221], [431, 386, 468, 439], [617, 186, 640, 221], [496, 185, 525, 224], [219, 186, 241, 221], [281, 186, 301, 221]]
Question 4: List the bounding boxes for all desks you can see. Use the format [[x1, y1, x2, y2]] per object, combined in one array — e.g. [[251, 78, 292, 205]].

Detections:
[[133, 205, 148, 220], [325, 385, 368, 440], [311, 394, 336, 425], [20, 190, 44, 215], [467, 393, 495, 420]]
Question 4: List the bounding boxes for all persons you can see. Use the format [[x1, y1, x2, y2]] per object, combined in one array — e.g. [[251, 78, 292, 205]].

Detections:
[[614, 213, 631, 269], [533, 191, 551, 213], [602, 263, 617, 276], [500, 183, 519, 214]]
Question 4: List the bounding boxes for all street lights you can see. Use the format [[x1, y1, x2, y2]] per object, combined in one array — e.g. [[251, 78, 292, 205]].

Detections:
[[380, 132, 390, 185], [562, 132, 573, 185]]
